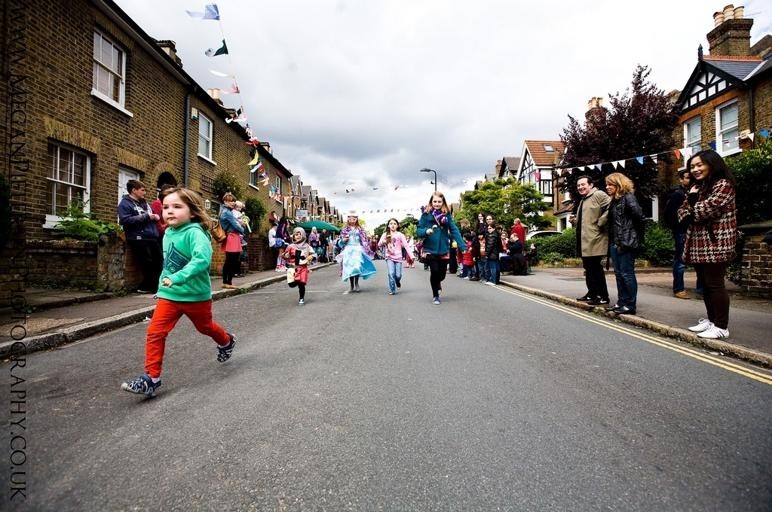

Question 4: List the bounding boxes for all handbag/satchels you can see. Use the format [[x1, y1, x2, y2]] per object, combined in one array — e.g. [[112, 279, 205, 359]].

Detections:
[[207, 219, 227, 243]]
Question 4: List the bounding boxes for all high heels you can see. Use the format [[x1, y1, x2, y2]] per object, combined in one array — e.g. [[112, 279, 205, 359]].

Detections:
[[350, 285, 360, 292]]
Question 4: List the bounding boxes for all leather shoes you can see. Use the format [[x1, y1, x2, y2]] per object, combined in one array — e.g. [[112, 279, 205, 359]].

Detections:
[[605, 303, 620, 311], [674, 290, 690, 299], [613, 306, 636, 315]]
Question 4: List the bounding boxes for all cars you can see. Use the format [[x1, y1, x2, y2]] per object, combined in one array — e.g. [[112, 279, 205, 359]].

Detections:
[[503, 230, 561, 246]]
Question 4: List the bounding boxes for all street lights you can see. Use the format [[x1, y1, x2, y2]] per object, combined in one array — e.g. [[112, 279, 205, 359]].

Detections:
[[419, 167, 439, 202]]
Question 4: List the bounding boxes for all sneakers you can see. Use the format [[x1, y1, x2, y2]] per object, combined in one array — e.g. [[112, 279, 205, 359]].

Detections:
[[118, 374, 162, 398], [216, 332, 236, 362], [587, 297, 610, 305], [388, 284, 401, 295], [577, 293, 594, 301], [688, 318, 714, 332], [697, 324, 730, 339], [457, 272, 496, 288], [298, 298, 305, 304], [433, 288, 442, 304]]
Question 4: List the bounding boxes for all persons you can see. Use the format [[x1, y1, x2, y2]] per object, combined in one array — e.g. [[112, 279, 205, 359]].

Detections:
[[665, 170, 703, 298], [119, 181, 249, 293], [677, 150, 737, 339], [568, 176, 609, 306], [605, 173, 645, 314], [270, 192, 528, 307], [116, 187, 234, 396]]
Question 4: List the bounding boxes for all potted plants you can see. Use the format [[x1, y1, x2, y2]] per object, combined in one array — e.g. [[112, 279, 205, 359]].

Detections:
[[243, 197, 267, 238]]
